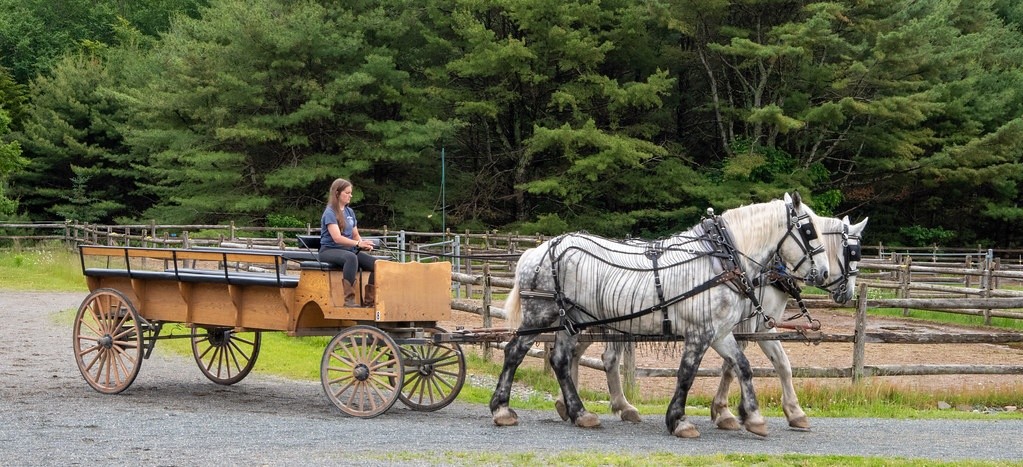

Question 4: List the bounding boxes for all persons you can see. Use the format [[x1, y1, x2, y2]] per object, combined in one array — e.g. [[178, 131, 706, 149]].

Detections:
[[319, 178, 377, 308]]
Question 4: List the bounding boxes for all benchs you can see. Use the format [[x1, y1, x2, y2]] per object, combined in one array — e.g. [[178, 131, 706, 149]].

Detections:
[[78, 246, 327, 289], [296, 234, 392, 269]]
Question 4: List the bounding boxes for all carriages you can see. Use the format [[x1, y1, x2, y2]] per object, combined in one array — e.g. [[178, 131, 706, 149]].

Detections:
[[72, 186, 869, 437]]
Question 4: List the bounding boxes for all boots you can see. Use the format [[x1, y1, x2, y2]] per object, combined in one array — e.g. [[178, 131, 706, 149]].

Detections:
[[362, 284, 375, 308], [342, 278, 361, 307]]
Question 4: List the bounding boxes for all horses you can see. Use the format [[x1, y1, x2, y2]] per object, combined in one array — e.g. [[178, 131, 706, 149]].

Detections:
[[556, 213, 868, 431], [490, 188, 828, 439]]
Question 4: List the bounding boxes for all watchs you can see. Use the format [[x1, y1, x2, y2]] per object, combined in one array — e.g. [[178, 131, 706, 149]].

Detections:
[[356, 241, 360, 249]]
